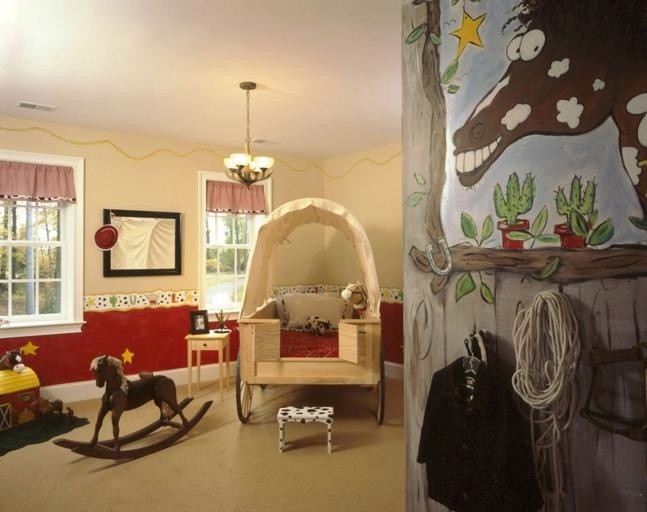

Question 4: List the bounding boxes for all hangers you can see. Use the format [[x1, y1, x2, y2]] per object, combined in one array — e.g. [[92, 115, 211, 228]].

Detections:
[[463, 335, 481, 375]]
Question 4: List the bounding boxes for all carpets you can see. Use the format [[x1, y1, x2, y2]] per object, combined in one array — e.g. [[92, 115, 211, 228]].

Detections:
[[0, 412, 90, 457]]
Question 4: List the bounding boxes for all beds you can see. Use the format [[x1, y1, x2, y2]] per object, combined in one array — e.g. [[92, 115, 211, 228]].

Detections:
[[235, 284, 385, 385]]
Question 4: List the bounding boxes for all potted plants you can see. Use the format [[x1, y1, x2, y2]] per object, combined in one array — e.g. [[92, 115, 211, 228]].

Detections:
[[494, 173, 536, 249], [553, 175, 597, 249]]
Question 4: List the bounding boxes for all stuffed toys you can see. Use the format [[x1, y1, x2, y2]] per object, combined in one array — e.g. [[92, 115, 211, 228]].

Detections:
[[0, 347, 24, 374], [339, 281, 367, 319], [302, 314, 337, 336]]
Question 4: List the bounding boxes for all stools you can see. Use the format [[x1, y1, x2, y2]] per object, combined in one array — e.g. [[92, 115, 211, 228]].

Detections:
[[277, 406, 334, 454]]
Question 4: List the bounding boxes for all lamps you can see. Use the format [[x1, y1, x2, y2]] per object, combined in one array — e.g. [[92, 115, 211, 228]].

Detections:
[[223, 81, 274, 190]]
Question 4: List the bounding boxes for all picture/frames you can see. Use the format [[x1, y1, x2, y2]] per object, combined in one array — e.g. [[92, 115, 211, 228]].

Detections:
[[189, 310, 210, 335], [102, 209, 181, 278]]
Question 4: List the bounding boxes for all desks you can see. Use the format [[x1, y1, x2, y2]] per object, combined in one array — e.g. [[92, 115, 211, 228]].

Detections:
[[184, 328, 232, 403]]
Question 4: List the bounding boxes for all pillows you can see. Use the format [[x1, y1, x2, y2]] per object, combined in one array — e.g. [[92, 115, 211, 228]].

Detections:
[[318, 283, 355, 330], [272, 285, 316, 325], [285, 292, 344, 332]]
